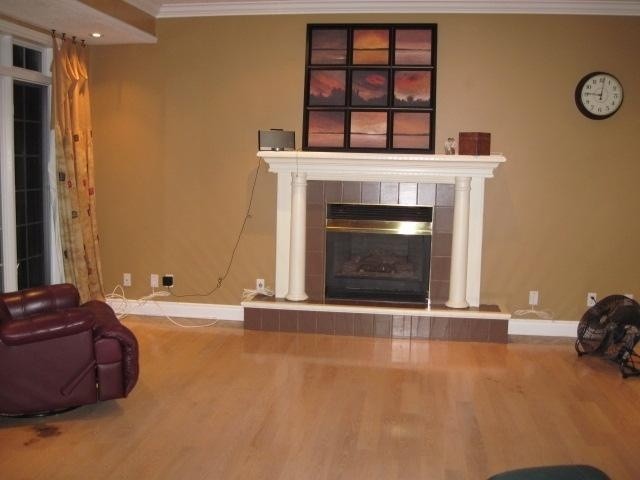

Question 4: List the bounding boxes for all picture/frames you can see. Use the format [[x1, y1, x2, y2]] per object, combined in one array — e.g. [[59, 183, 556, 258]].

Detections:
[[299, 19, 439, 158]]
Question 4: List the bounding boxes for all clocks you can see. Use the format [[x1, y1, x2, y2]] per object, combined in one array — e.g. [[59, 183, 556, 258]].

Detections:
[[575, 72, 625, 123]]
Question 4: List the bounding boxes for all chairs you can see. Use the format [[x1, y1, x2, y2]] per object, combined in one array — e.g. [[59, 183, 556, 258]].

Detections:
[[0, 280, 134, 416]]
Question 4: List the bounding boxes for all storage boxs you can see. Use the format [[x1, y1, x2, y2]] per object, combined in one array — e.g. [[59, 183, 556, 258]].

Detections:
[[457, 130, 493, 156]]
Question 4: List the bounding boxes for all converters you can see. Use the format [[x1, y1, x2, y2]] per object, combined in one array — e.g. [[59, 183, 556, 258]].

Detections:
[[163, 277, 173, 286]]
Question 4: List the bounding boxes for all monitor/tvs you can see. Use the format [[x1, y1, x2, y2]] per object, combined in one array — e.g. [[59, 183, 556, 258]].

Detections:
[[258, 129, 295, 151]]
